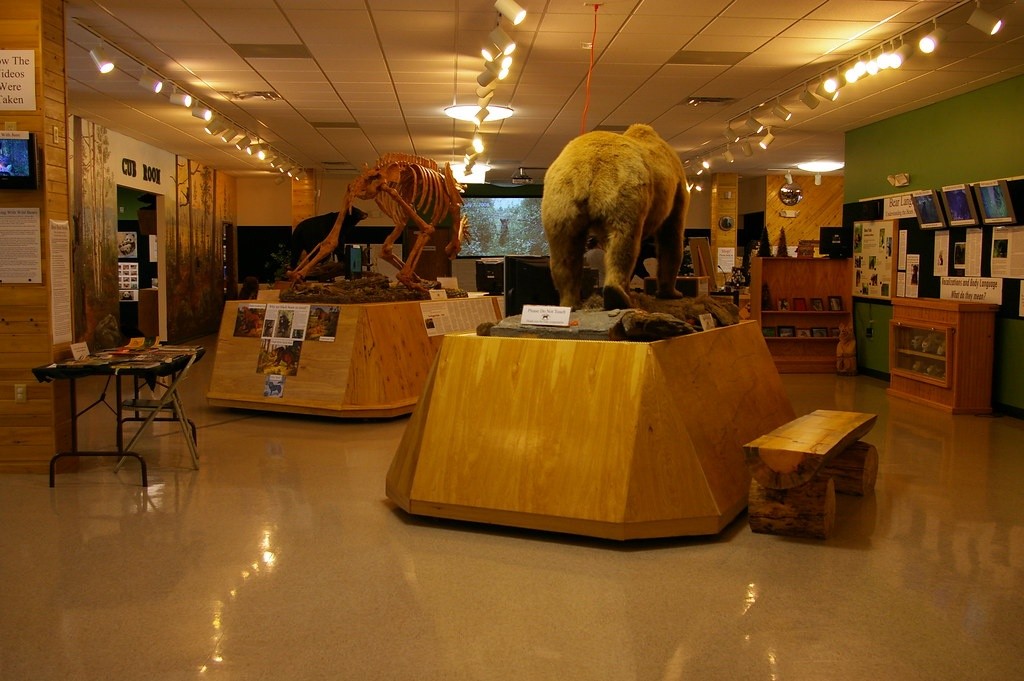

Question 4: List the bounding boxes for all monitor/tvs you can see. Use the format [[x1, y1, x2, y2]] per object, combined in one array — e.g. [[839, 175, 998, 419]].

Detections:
[[820, 226, 853, 258], [476, 258, 505, 296], [344, 244, 363, 280], [0, 131, 39, 189], [910, 180, 1017, 230]]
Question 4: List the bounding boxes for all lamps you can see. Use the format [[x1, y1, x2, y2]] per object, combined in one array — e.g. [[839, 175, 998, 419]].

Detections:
[[246, 144, 263, 155], [257, 150, 274, 161], [192, 106, 212, 121], [279, 162, 292, 173], [463, 0, 527, 177], [270, 158, 283, 168], [295, 171, 307, 181], [204, 118, 227, 136], [688, 0, 1002, 192], [138, 76, 163, 94], [90, 47, 115, 74], [221, 129, 238, 143], [235, 136, 251, 151], [886, 173, 910, 187], [287, 168, 299, 178], [169, 93, 192, 108]]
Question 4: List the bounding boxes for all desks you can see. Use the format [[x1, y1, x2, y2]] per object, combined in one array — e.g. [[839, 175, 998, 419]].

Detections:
[[31, 342, 207, 489]]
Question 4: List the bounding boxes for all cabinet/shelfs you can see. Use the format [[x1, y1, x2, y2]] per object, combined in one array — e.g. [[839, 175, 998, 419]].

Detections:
[[750, 256, 853, 374], [138, 288, 159, 338], [886, 296, 1000, 415]]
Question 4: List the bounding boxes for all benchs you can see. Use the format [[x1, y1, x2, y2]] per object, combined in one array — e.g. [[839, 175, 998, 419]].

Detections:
[[742, 407, 879, 541]]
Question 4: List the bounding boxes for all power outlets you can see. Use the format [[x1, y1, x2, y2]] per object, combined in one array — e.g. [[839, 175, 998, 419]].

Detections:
[[15, 384, 27, 404]]
